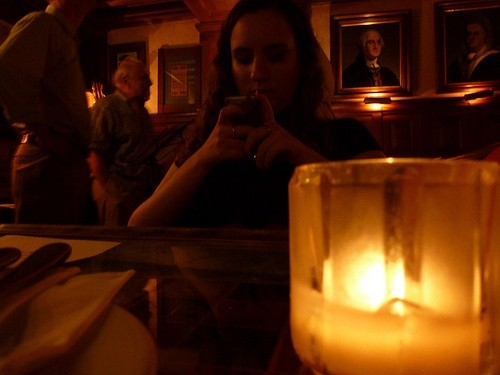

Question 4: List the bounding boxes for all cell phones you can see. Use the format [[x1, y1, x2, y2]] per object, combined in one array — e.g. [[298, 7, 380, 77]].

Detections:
[[225, 97, 264, 150]]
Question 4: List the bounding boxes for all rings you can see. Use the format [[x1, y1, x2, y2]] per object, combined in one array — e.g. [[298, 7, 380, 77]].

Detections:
[[232, 126, 235, 136]]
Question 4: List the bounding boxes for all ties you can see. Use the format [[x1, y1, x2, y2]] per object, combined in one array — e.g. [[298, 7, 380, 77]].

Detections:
[[371, 67, 382, 87]]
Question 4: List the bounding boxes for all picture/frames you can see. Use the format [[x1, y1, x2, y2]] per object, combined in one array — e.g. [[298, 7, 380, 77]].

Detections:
[[109, 41, 146, 84], [432, 0, 500, 88], [158, 47, 201, 114], [330, 9, 413, 95]]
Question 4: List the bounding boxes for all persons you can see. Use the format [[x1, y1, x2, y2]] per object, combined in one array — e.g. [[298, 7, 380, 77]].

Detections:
[[89, 59, 156, 225], [0, 0, 93, 225], [139, 241, 290, 366], [128, 0, 385, 227]]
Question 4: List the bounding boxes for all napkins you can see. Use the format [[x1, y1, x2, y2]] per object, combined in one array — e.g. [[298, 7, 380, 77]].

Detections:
[[0, 269, 134, 375]]
